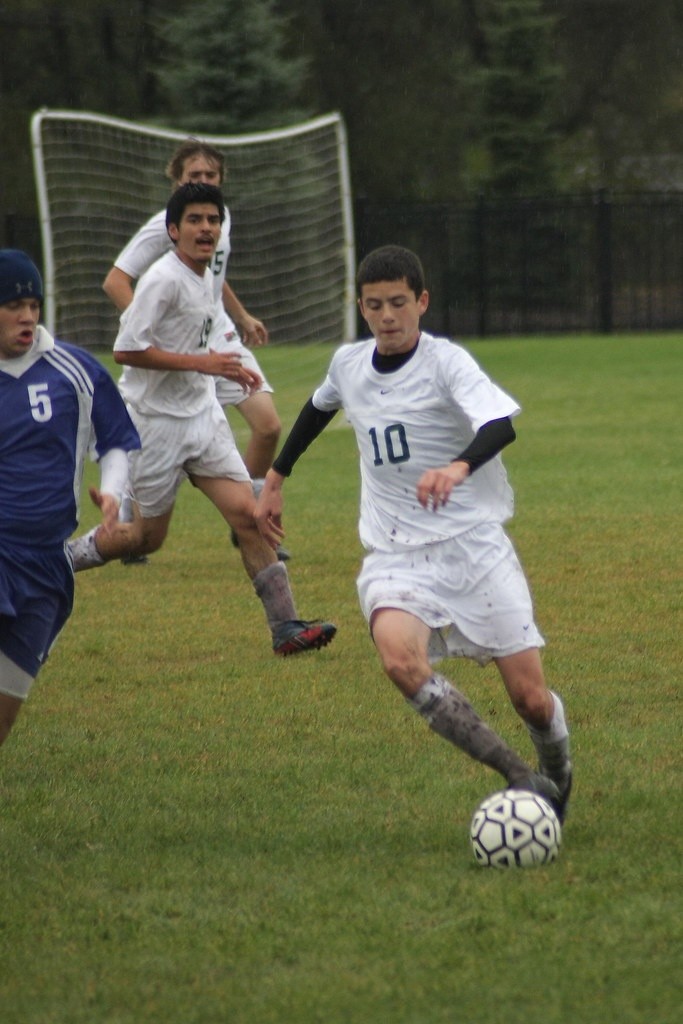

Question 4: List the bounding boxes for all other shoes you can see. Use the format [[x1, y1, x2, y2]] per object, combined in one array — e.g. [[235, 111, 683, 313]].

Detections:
[[230, 528, 292, 561], [510, 776, 562, 804], [121, 554, 147, 564], [534, 760, 574, 821]]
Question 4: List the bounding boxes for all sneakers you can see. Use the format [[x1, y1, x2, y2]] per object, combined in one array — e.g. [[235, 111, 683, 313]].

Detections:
[[273, 618, 336, 655]]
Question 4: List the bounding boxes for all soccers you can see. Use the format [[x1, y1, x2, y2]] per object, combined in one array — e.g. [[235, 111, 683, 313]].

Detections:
[[467, 787, 564, 873]]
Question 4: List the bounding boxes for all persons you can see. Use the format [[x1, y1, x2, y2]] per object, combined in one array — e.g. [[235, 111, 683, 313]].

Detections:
[[251, 244, 573, 827], [0, 247, 130, 747], [66, 142, 338, 657]]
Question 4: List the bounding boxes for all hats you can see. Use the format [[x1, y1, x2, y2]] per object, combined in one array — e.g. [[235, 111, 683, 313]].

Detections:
[[0, 250, 43, 307]]
